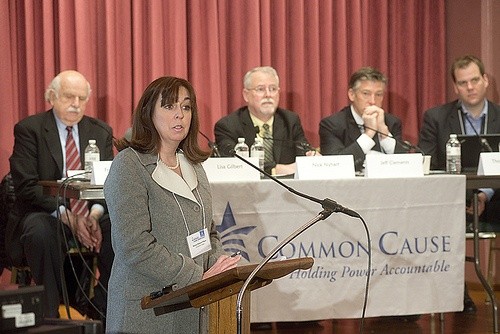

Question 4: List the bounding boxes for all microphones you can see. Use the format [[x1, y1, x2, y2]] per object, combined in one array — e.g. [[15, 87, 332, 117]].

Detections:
[[255, 126, 316, 150], [458, 104, 493, 153], [217, 139, 360, 218], [348, 119, 425, 164], [90, 117, 117, 140]]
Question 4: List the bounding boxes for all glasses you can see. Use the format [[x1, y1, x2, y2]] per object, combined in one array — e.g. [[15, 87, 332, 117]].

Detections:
[[246, 84, 279, 94]]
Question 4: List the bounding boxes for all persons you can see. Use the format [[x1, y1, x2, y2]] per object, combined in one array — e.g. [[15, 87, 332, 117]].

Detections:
[[214, 66, 322, 334], [103, 76, 242, 334], [318, 66, 423, 334], [9, 69, 116, 328], [417, 56, 500, 334]]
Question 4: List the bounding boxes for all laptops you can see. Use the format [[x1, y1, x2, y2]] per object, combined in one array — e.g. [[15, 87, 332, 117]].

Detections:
[[458, 134, 500, 173]]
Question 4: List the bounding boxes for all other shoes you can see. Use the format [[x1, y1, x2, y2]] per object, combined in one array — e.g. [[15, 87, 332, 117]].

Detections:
[[400, 315, 420, 323], [454, 289, 476, 314], [251, 323, 271, 330]]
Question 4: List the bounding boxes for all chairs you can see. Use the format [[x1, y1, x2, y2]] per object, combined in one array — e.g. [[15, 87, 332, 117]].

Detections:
[[0, 170, 98, 320]]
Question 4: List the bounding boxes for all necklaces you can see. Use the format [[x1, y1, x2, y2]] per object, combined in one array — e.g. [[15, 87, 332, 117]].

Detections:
[[168, 158, 179, 170]]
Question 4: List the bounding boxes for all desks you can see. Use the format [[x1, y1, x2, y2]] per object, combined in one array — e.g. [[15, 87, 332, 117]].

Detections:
[[37, 171, 500, 334]]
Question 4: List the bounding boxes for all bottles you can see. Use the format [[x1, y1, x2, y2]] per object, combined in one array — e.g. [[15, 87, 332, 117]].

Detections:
[[250, 137, 264, 176], [234, 137, 249, 159], [446, 133, 462, 174], [84, 139, 100, 179]]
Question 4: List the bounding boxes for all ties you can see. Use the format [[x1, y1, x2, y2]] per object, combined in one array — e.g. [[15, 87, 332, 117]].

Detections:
[[262, 124, 274, 167], [65, 125, 89, 220]]
